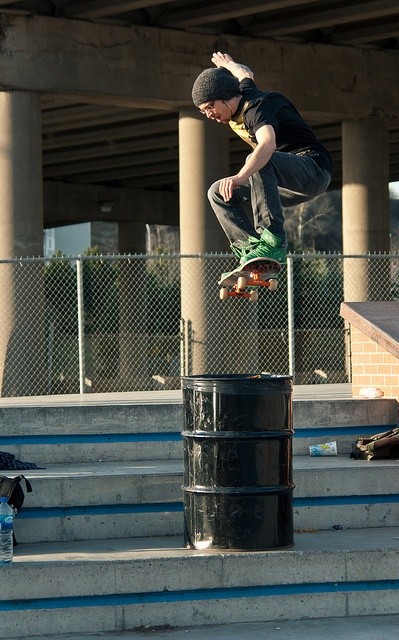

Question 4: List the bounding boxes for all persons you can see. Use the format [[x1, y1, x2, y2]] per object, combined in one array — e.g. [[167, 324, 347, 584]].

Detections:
[[190, 49, 335, 290]]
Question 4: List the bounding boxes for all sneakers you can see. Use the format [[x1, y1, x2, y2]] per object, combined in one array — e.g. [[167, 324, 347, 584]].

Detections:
[[229, 237, 258, 260], [239, 228, 287, 265]]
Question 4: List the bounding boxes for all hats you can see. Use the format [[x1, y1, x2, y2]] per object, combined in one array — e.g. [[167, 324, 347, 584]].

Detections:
[[192, 66, 240, 107]]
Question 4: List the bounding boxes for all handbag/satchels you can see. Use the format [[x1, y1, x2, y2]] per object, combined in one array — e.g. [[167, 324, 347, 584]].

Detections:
[[0, 474, 32, 548], [350, 427, 399, 460]]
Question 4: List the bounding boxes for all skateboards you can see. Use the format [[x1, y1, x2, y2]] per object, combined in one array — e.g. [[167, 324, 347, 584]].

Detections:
[[217, 257, 281, 303]]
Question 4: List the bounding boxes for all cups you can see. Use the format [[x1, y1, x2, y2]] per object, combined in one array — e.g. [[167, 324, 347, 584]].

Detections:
[[308, 441, 338, 456]]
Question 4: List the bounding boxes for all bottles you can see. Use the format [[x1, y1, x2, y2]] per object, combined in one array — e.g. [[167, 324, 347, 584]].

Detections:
[[0, 497, 15, 563]]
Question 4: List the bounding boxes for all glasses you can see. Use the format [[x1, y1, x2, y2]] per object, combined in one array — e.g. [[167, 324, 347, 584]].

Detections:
[[199, 99, 215, 115]]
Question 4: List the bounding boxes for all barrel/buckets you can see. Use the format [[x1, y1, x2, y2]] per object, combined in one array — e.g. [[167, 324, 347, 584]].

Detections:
[[177, 372, 294, 551]]
